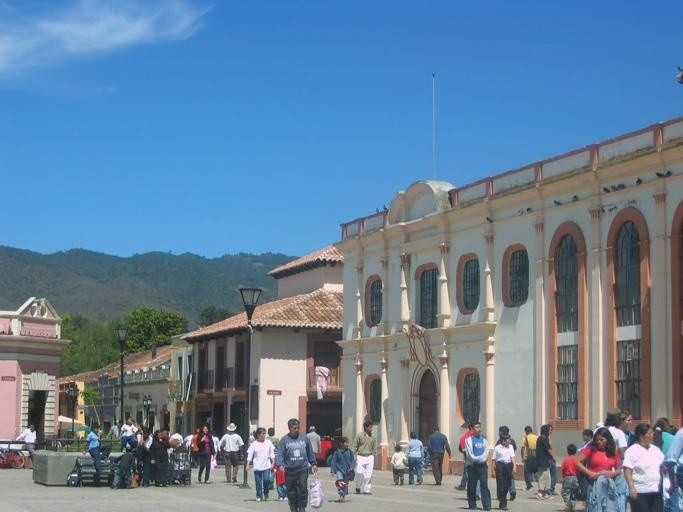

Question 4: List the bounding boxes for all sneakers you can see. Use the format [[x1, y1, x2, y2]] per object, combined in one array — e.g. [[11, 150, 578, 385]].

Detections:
[[392, 479, 423, 484], [470, 496, 507, 508], [524, 485, 532, 491], [455, 486, 466, 490], [356, 488, 371, 495], [206, 480, 212, 483], [256, 496, 269, 501]]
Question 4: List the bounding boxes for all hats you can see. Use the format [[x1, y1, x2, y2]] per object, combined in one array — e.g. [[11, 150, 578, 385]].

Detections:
[[606, 409, 622, 419], [227, 422, 237, 431]]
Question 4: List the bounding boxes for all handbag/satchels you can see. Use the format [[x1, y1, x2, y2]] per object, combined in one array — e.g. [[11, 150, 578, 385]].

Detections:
[[310, 481, 323, 507], [213, 459, 217, 467], [527, 455, 537, 471], [577, 474, 590, 492], [350, 469, 355, 481]]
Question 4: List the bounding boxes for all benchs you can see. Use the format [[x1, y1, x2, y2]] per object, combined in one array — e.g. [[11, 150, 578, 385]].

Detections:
[[75, 457, 120, 486]]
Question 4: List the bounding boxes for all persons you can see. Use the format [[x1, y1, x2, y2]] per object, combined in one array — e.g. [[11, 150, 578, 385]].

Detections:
[[277, 419, 318, 512], [352, 420, 377, 495], [521, 424, 557, 499], [405, 432, 424, 485], [560, 409, 683, 512], [427, 424, 451, 485], [331, 437, 354, 502], [246, 428, 288, 501], [391, 444, 407, 485], [87, 416, 219, 488], [13, 423, 37, 461], [492, 426, 517, 510], [219, 423, 244, 482], [305, 425, 322, 474], [456, 421, 491, 511]]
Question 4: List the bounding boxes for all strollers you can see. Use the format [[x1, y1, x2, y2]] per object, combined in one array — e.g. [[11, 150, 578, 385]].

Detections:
[[170, 446, 192, 486]]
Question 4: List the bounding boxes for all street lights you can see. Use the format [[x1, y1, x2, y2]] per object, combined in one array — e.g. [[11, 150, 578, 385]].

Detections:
[[116, 324, 130, 426], [67, 382, 80, 440], [239, 288, 262, 489], [144, 394, 152, 429]]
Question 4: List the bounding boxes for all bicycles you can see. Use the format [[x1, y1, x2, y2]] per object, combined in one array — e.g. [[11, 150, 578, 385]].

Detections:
[[1, 441, 25, 469]]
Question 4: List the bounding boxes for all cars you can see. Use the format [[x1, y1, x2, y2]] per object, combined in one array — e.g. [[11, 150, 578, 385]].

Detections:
[[316, 435, 341, 466]]
